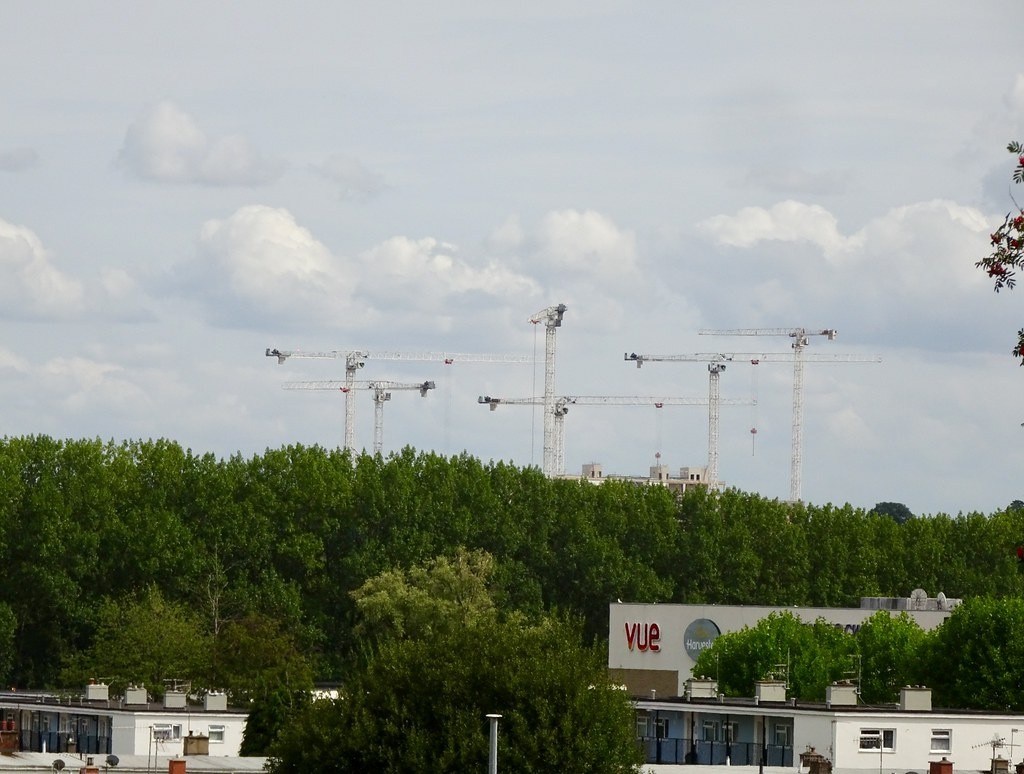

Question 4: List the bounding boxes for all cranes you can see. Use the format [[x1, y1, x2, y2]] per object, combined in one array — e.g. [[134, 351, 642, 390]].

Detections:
[[697, 327, 838, 501], [526, 302, 567, 474], [263, 346, 544, 468], [623, 351, 884, 492], [280, 378, 436, 458], [476, 394, 759, 479]]
